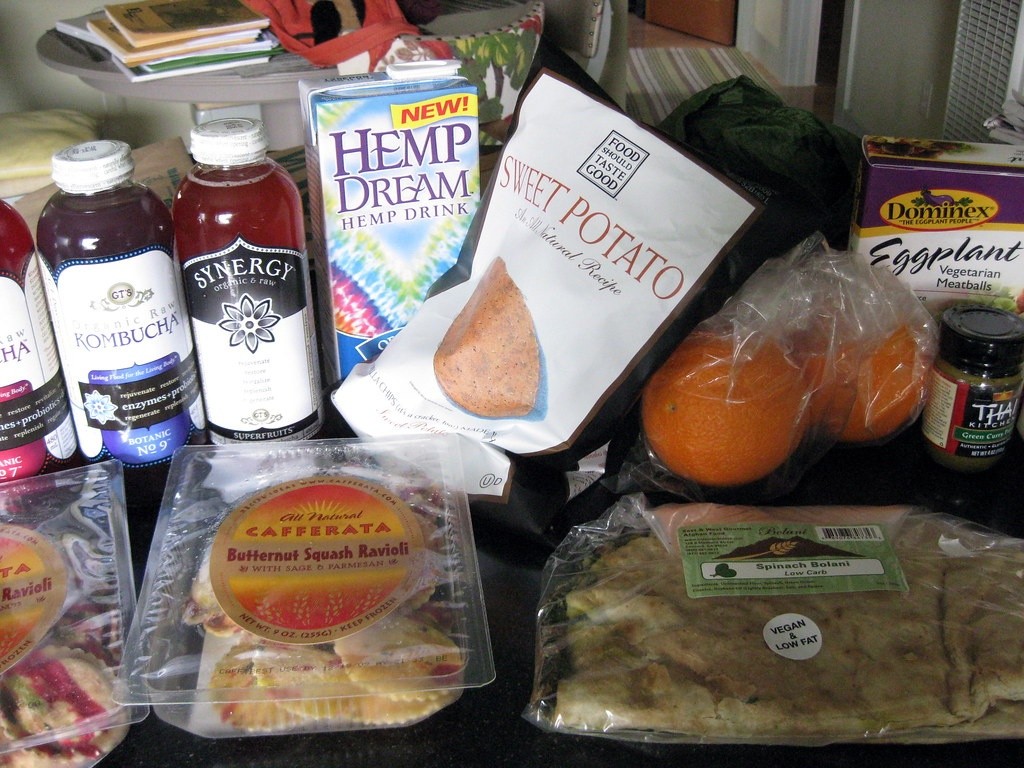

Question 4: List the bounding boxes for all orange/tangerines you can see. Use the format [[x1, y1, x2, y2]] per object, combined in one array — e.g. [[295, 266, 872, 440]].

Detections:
[[786, 304, 932, 442], [642, 324, 810, 486]]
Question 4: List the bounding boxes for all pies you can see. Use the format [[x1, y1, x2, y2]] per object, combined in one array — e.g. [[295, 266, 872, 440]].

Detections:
[[545, 514, 1024, 739]]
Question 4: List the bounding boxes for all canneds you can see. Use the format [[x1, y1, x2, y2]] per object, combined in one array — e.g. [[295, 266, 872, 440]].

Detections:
[[921, 304, 1024, 473]]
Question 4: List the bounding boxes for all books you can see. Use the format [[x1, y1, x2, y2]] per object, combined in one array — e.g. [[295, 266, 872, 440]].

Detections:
[[55, 0, 285, 84]]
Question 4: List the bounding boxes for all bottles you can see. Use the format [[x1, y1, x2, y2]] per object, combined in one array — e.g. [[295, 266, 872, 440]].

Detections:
[[0, 196, 88, 525], [920, 303, 1024, 469], [172, 116, 325, 459], [37, 139, 208, 509]]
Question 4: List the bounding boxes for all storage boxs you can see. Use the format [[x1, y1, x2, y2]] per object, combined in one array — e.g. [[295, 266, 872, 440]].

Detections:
[[299, 61, 479, 386], [845, 134, 1024, 323]]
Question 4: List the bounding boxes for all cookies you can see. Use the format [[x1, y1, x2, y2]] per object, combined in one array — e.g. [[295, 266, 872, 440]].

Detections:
[[432, 255, 541, 419]]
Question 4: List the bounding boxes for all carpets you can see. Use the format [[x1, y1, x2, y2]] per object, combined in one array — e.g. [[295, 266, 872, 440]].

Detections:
[[624, 47, 784, 128]]
